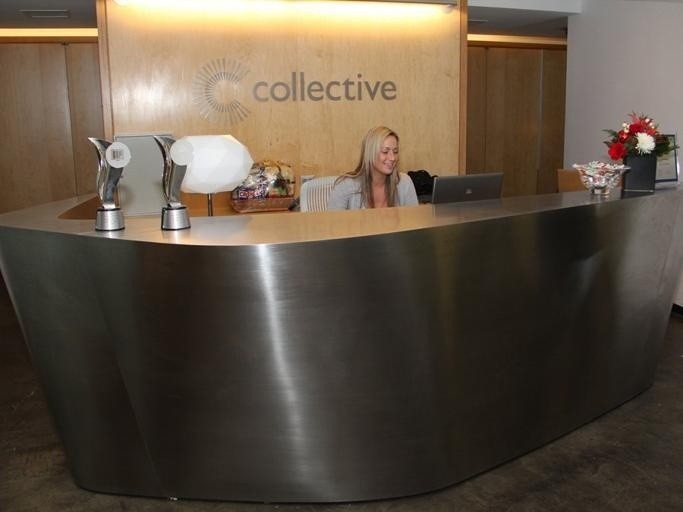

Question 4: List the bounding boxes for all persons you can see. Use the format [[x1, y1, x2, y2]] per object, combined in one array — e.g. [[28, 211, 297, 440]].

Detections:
[[325, 126, 419, 211]]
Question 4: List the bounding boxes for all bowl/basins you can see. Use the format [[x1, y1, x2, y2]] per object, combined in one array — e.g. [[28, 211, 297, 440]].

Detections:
[[572, 161, 633, 197]]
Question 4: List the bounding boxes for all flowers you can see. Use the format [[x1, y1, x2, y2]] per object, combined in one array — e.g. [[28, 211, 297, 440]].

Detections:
[[598, 110, 680, 161]]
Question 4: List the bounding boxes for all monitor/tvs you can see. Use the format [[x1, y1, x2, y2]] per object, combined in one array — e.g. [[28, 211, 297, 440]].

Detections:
[[432, 172, 504, 204]]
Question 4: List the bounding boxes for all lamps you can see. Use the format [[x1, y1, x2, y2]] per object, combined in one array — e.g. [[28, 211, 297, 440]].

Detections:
[[178, 134, 254, 216]]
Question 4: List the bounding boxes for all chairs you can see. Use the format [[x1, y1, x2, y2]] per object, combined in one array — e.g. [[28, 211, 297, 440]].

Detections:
[[299, 175, 348, 212]]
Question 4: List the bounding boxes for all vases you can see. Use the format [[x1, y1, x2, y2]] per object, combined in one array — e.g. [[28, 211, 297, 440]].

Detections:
[[622, 153, 657, 193]]
